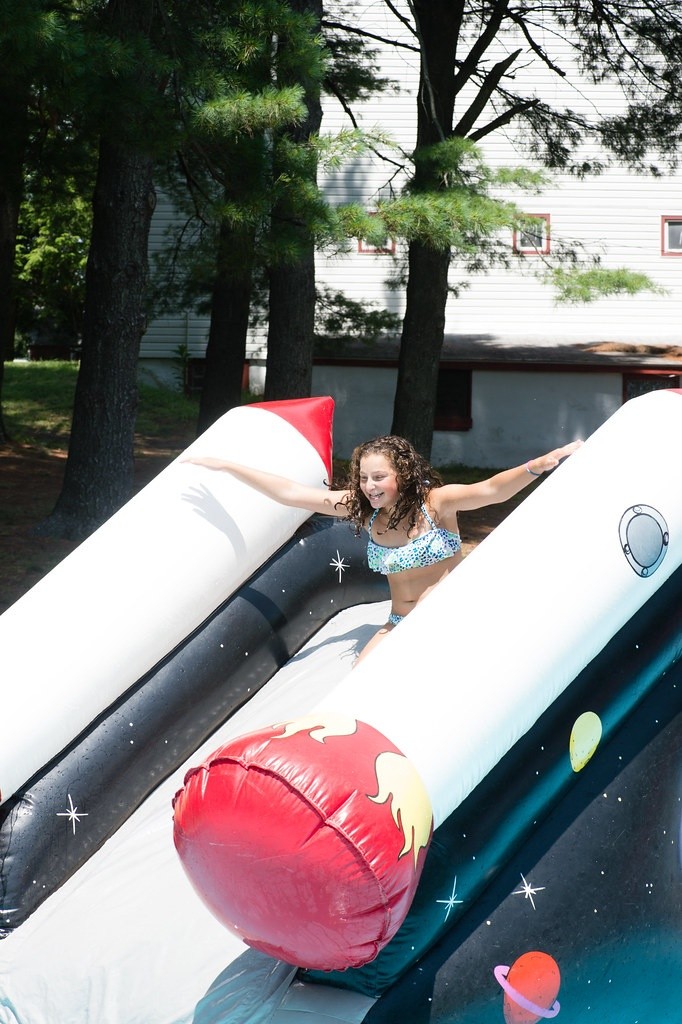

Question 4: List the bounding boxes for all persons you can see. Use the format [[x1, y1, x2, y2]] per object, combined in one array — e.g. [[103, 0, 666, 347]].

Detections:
[[181, 435, 580, 669]]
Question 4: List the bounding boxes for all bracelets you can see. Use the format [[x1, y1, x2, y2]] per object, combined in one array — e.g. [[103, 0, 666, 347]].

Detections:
[[527, 459, 543, 476]]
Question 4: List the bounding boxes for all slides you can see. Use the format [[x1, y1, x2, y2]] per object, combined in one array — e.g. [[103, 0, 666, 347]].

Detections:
[[0, 386, 681, 1024]]
